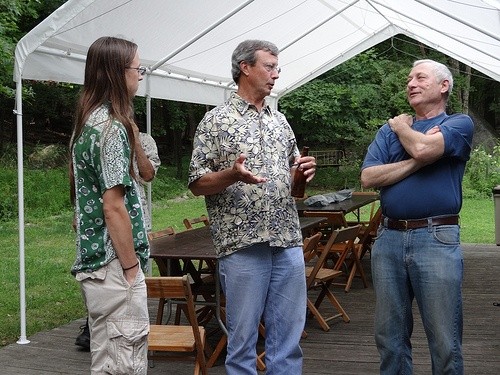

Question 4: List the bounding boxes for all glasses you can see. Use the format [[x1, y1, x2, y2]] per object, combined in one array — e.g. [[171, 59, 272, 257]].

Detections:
[[264, 63, 281, 75], [124, 66, 146, 75]]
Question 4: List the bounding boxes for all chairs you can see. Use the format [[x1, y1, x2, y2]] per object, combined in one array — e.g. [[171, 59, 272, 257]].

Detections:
[[303, 206, 388, 338], [145, 213, 266, 375]]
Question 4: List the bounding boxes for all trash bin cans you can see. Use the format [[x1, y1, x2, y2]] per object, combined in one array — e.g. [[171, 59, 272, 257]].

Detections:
[[492, 185, 500, 246]]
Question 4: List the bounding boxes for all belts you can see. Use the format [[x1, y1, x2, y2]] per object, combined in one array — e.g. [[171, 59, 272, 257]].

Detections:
[[381, 215, 460, 231]]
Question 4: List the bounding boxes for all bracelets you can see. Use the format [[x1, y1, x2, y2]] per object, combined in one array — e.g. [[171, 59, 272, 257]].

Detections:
[[122, 262, 139, 274]]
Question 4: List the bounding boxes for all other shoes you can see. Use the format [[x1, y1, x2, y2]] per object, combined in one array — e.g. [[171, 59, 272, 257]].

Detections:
[[74, 316, 90, 347]]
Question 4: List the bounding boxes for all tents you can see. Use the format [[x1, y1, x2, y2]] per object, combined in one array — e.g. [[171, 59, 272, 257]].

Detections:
[[12, 1, 500, 344]]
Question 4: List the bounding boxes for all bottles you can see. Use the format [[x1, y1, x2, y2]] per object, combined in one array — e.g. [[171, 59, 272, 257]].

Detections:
[[290, 146, 309, 198]]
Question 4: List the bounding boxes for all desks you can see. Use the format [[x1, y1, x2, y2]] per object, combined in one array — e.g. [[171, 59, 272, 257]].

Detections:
[[149, 218, 327, 368], [296, 192, 381, 262]]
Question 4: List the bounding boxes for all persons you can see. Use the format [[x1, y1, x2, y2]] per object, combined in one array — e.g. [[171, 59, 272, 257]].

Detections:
[[360, 59, 476, 375], [67, 37, 157, 375], [72, 106, 161, 350], [187, 40, 316, 375]]
